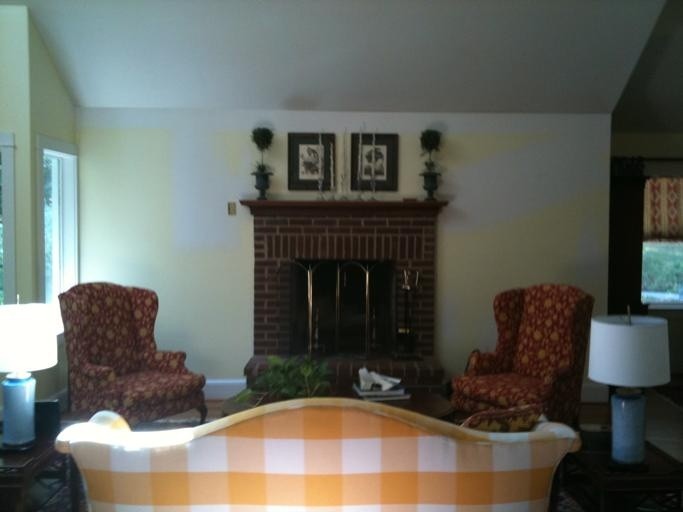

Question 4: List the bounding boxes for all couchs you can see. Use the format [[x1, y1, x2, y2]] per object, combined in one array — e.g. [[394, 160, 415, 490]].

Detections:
[[54, 397, 583, 512], [58, 281, 208, 426], [450, 283, 595, 428]]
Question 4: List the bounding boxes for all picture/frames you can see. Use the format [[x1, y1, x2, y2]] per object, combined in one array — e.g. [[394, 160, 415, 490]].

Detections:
[[287, 132, 336, 191], [350, 132, 399, 192]]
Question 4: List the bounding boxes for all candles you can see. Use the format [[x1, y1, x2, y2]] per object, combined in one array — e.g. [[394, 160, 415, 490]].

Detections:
[[330, 143, 334, 189], [357, 126, 362, 182], [318, 133, 322, 177], [343, 129, 347, 180], [371, 131, 376, 182]]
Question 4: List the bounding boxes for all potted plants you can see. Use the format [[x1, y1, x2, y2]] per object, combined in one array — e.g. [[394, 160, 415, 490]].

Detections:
[[250, 127, 275, 200], [417, 129, 442, 202]]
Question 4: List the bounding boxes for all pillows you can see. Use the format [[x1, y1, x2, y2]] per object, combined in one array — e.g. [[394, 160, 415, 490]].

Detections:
[[460, 400, 545, 433]]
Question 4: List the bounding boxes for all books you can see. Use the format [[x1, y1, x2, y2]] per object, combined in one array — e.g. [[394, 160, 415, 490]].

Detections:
[[352, 383, 411, 401]]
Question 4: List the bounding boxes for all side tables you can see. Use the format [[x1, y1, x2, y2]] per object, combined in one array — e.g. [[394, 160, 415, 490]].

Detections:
[[0, 436, 57, 512], [557, 427, 683, 512]]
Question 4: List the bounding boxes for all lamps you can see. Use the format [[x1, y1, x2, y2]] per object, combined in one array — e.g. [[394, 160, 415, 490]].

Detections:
[[0, 294, 59, 452], [587, 305, 671, 465]]
